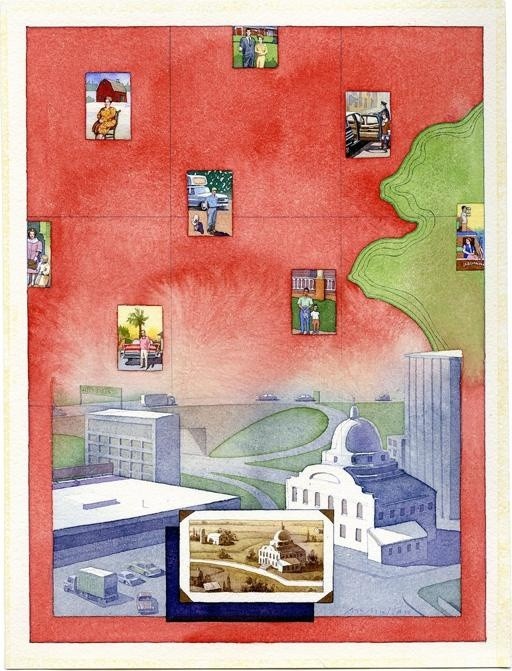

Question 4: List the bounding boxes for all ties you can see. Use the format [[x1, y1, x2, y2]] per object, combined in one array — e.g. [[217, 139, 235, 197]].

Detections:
[[248, 38, 251, 46]]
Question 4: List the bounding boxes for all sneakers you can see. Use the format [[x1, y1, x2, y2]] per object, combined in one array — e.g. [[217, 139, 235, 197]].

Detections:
[[378, 146, 383, 149]]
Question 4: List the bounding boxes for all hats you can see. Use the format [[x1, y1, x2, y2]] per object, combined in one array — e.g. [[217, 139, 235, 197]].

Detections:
[[380, 101, 387, 105], [212, 188, 217, 191]]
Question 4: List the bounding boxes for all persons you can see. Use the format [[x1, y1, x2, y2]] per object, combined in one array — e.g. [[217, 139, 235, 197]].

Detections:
[[35, 255, 50, 285], [309, 305, 320, 334], [205, 188, 218, 234], [238, 28, 256, 68], [28, 227, 42, 286], [463, 239, 478, 260], [368, 102, 390, 124], [139, 330, 151, 369], [460, 206, 470, 231], [380, 112, 390, 152], [254, 35, 267, 68], [297, 288, 313, 335], [94, 96, 118, 139]]
